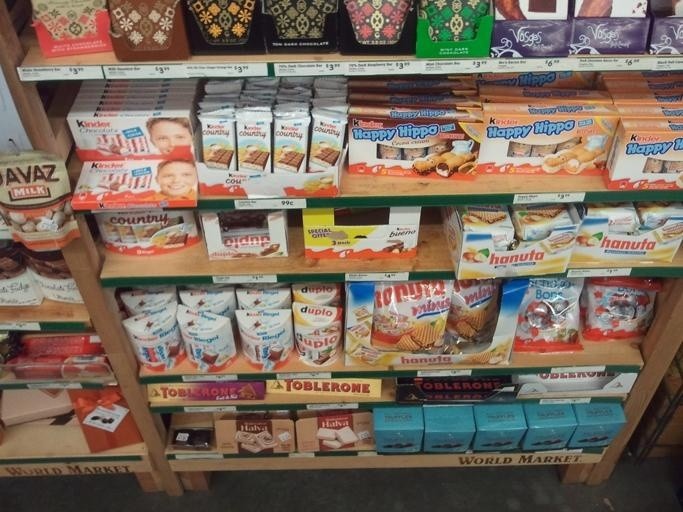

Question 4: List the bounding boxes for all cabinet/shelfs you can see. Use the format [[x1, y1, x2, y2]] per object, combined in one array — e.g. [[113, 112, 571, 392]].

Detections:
[[1, 0, 683, 498], [0, 1, 183, 498], [587, 276, 683, 488]]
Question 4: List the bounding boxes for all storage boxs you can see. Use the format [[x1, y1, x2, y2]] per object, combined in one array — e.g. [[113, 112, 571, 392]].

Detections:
[[134, 473, 211, 492]]
